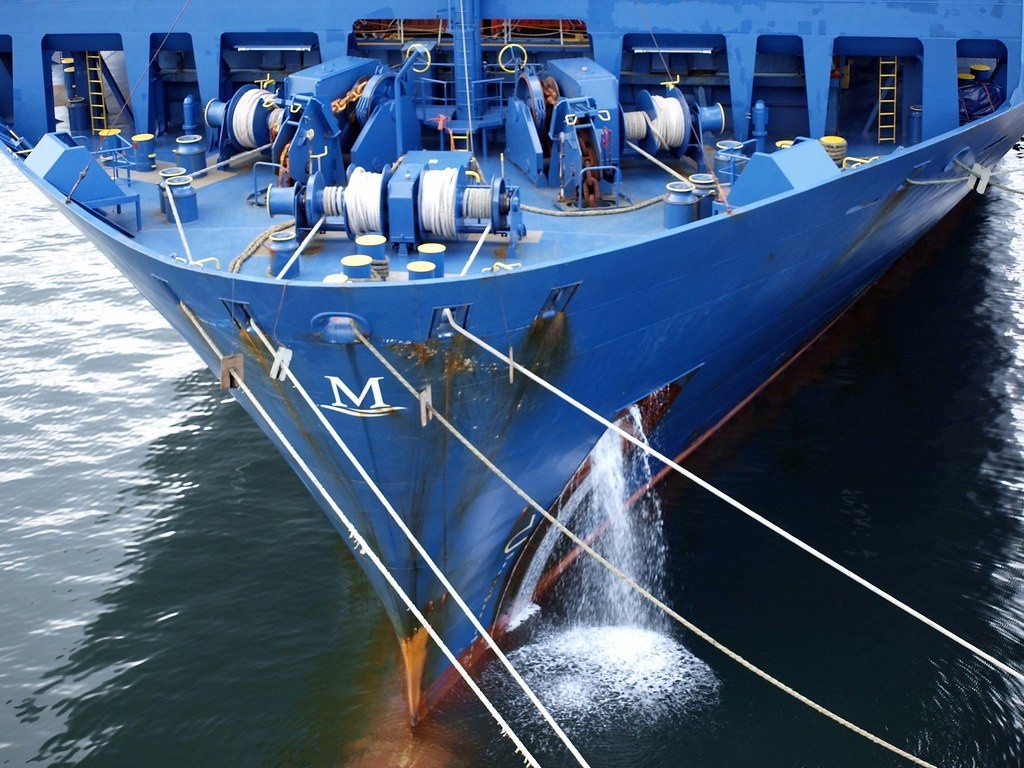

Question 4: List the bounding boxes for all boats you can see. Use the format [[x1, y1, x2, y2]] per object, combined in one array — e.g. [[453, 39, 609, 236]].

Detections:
[[0, 1, 1024, 740]]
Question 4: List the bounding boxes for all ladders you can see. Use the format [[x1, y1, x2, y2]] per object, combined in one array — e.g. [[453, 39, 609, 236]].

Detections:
[[878, 56, 897, 144], [86, 51, 107, 135]]
